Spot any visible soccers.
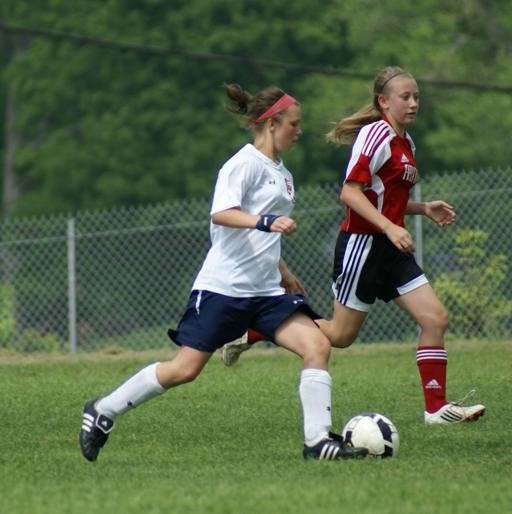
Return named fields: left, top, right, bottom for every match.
left=343, top=410, right=401, bottom=461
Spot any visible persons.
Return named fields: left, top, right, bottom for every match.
left=79, top=81, right=369, bottom=464
left=218, top=64, right=487, bottom=426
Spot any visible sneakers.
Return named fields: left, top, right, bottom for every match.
left=303, top=431, right=369, bottom=461
left=423, top=402, right=486, bottom=427
left=222, top=330, right=249, bottom=367
left=79, top=396, right=114, bottom=463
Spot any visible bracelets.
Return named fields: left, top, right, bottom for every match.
left=256, top=213, right=280, bottom=231
